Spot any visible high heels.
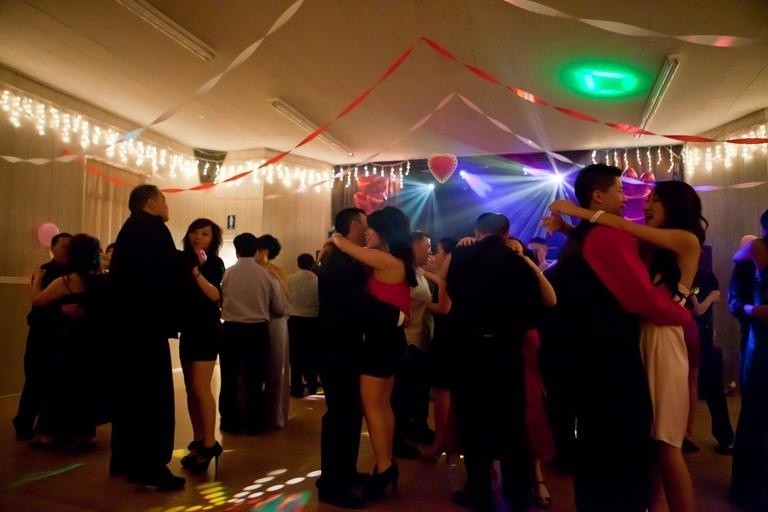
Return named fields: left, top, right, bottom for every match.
left=189, top=442, right=223, bottom=473
left=421, top=434, right=457, bottom=466
left=181, top=440, right=201, bottom=464
left=364, top=462, right=400, bottom=497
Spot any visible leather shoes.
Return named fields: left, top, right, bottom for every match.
left=127, top=466, right=186, bottom=491
left=683, top=432, right=700, bottom=453
left=345, top=471, right=371, bottom=487
left=535, top=480, right=551, bottom=507
left=718, top=432, right=735, bottom=454
left=391, top=436, right=420, bottom=458
left=318, top=490, right=367, bottom=509
left=451, top=489, right=488, bottom=510
left=13, top=417, right=97, bottom=455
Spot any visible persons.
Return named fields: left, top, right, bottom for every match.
left=287, top=161, right=766, bottom=512
left=11, top=185, right=287, bottom=488
